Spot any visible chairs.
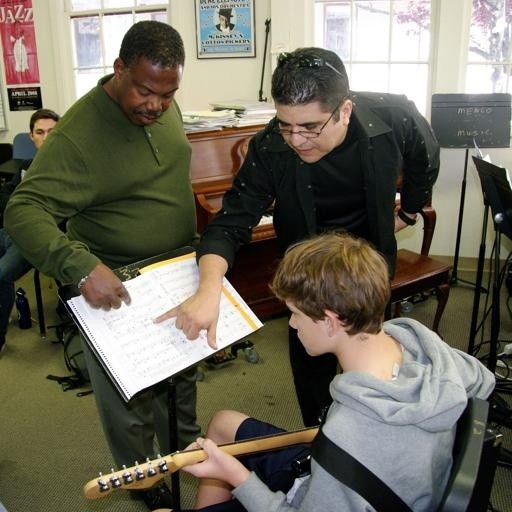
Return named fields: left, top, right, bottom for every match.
left=438, top=397, right=491, bottom=512
left=0, top=134, right=65, bottom=337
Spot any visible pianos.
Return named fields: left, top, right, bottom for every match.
left=184, top=123, right=436, bottom=321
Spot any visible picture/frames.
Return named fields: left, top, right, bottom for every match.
left=194, top=0, right=256, bottom=59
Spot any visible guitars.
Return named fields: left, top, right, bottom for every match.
left=81, top=424, right=321, bottom=499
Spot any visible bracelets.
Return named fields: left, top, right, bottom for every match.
left=397, top=210, right=418, bottom=229
left=76, top=274, right=90, bottom=290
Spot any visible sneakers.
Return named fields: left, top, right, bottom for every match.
left=139, top=483, right=174, bottom=509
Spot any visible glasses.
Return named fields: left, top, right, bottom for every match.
left=275, top=52, right=346, bottom=79
left=272, top=98, right=347, bottom=137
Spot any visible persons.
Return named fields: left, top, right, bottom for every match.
left=2, top=18, right=201, bottom=509
left=154, top=44, right=442, bottom=432
left=179, top=230, right=496, bottom=511
left=216, top=6, right=237, bottom=34
left=2, top=106, right=70, bottom=352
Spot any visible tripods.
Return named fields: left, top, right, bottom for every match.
left=486, top=213, right=512, bottom=428
left=431, top=149, right=489, bottom=293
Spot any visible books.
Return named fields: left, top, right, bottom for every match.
left=179, top=99, right=277, bottom=135
left=67, top=257, right=265, bottom=401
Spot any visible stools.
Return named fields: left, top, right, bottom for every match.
left=385, top=250, right=452, bottom=340
